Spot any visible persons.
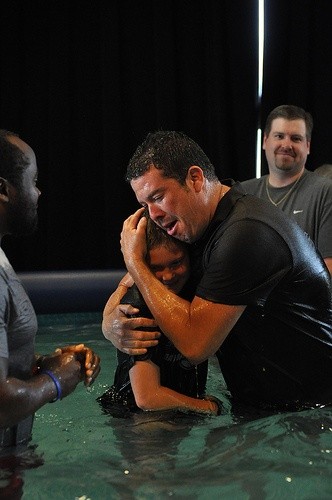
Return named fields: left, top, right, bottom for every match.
left=97, top=209, right=225, bottom=465
left=0, top=129, right=101, bottom=458
left=239, top=105, right=332, bottom=277
left=102, top=129, right=332, bottom=423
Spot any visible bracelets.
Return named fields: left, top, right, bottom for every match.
left=39, top=370, right=63, bottom=404
